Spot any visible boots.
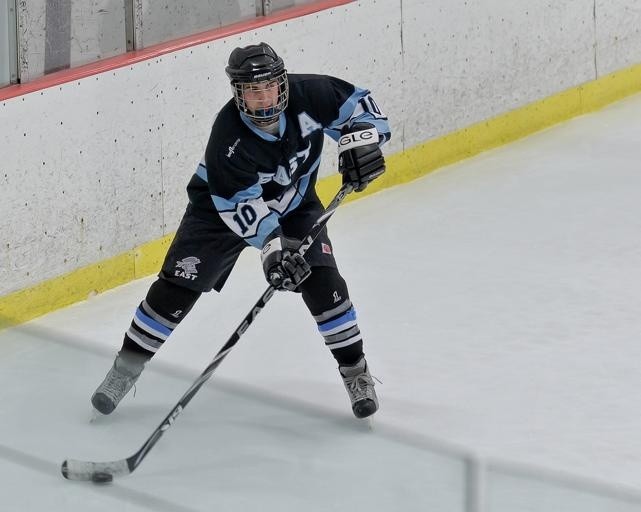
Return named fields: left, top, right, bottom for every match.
left=338, top=353, right=380, bottom=420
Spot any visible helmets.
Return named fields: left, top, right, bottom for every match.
left=225, top=41, right=284, bottom=82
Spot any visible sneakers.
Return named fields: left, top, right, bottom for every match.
left=91, top=356, right=145, bottom=415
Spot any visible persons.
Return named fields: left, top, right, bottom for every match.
left=91, top=39, right=393, bottom=420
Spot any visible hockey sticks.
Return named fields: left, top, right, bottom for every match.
left=62, top=176, right=355, bottom=482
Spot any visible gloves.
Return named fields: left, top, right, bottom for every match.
left=260, top=225, right=312, bottom=292
left=338, top=122, right=386, bottom=192
